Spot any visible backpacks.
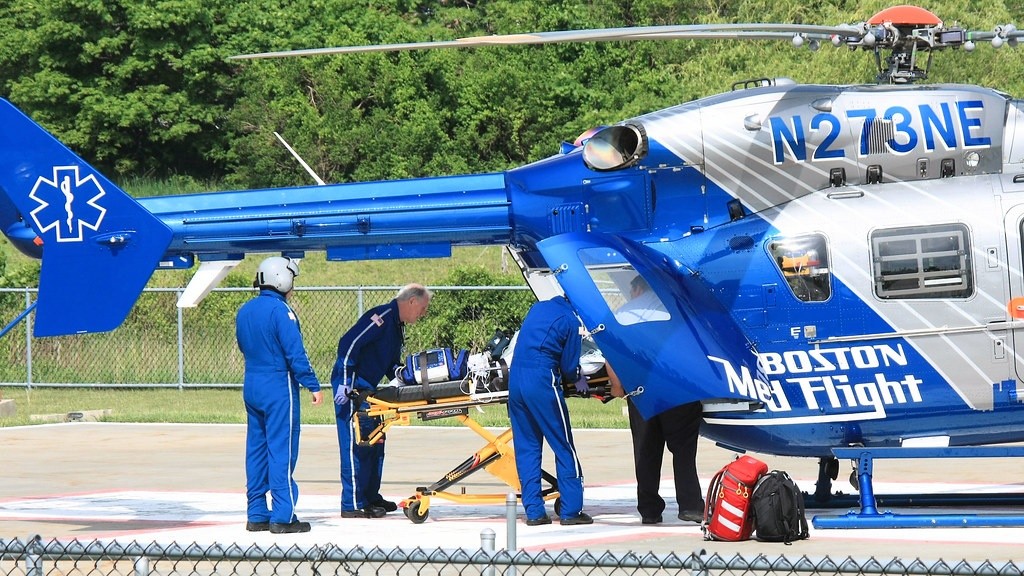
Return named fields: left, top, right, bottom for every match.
left=751, top=470, right=809, bottom=541
left=701, top=455, right=767, bottom=542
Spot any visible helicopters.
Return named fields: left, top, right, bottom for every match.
left=0, top=4, right=1024, bottom=529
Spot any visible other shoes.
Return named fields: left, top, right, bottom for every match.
left=641, top=515, right=662, bottom=524
left=247, top=521, right=268, bottom=531
left=678, top=509, right=711, bottom=523
left=270, top=520, right=311, bottom=533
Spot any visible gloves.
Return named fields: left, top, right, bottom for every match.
left=333, top=384, right=353, bottom=405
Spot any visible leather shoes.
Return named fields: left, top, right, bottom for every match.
left=560, top=513, right=594, bottom=525
left=341, top=505, right=385, bottom=518
left=527, top=514, right=552, bottom=525
left=372, top=499, right=397, bottom=512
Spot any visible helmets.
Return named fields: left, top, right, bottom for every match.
left=253, top=256, right=299, bottom=293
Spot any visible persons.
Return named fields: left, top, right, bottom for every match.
left=234, top=256, right=323, bottom=534
left=330, top=282, right=431, bottom=519
left=604, top=351, right=709, bottom=524
left=508, top=293, right=594, bottom=526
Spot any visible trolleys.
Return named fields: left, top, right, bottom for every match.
left=344, top=374, right=616, bottom=525
